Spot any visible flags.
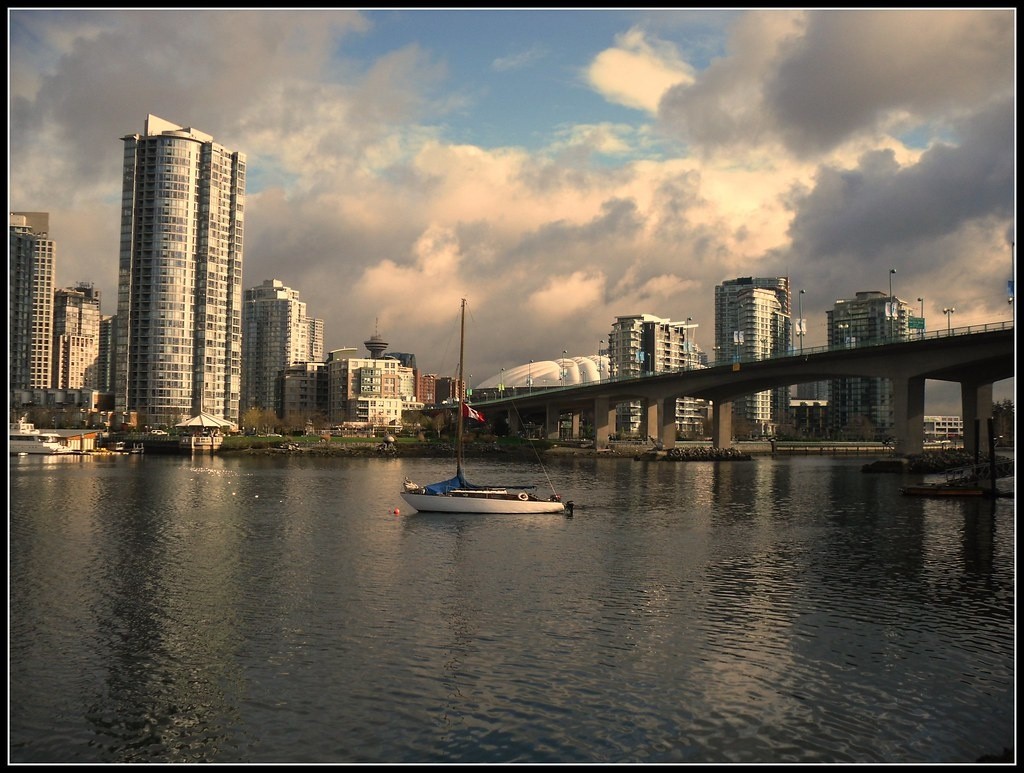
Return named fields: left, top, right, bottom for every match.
left=463, top=402, right=485, bottom=422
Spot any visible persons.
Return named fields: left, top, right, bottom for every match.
left=666, top=446, right=742, bottom=458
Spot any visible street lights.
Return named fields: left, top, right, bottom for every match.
left=761, top=339, right=767, bottom=360
left=838, top=321, right=849, bottom=349
left=501, top=368, right=505, bottom=400
left=561, top=350, right=567, bottom=386
left=1008, top=296, right=1014, bottom=322
left=889, top=269, right=897, bottom=342
left=529, top=360, right=533, bottom=396
left=799, top=289, right=806, bottom=355
left=712, top=346, right=720, bottom=366
left=581, top=370, right=586, bottom=385
left=686, top=317, right=692, bottom=371
left=600, top=340, right=604, bottom=384
left=917, top=298, right=924, bottom=340
left=737, top=304, right=743, bottom=363
left=943, top=307, right=955, bottom=336
left=638, top=329, right=644, bottom=379
left=468, top=375, right=472, bottom=404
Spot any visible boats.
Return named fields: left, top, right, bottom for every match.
left=10, top=416, right=73, bottom=454
left=899, top=416, right=1014, bottom=497
left=107, top=441, right=144, bottom=454
left=399, top=298, right=574, bottom=514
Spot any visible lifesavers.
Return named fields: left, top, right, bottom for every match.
left=518, top=492, right=528, bottom=501
left=668, top=447, right=741, bottom=459
